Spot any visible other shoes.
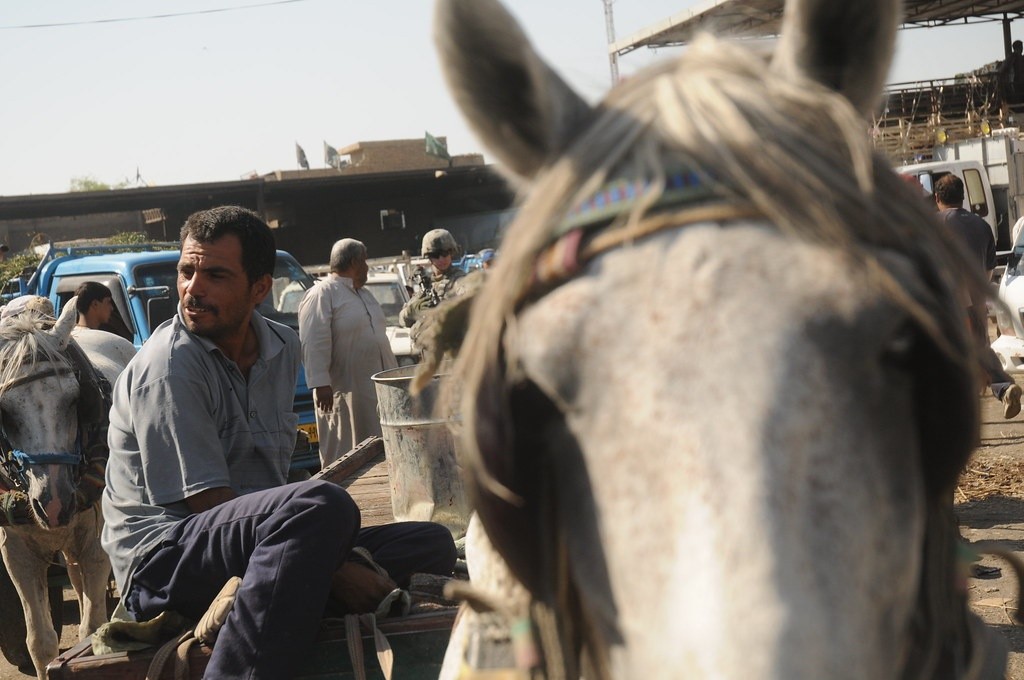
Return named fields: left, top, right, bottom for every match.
left=1000, top=384, right=1022, bottom=419
left=193, top=576, right=243, bottom=648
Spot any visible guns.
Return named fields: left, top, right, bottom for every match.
left=410, top=267, right=441, bottom=307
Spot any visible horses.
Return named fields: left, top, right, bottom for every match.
left=0, top=292, right=138, bottom=680
left=420, top=0, right=1007, bottom=679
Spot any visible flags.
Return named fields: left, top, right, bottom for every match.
left=425, top=135, right=452, bottom=160
left=324, top=144, right=342, bottom=174
left=296, top=145, right=310, bottom=171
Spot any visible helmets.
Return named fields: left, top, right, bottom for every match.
left=479, top=249, right=495, bottom=268
left=421, top=228, right=458, bottom=259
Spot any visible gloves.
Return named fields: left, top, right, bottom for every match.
left=404, top=290, right=434, bottom=320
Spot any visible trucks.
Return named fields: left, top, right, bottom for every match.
left=0, top=240, right=321, bottom=480
left=320, top=254, right=502, bottom=366
left=890, top=134, right=1024, bottom=275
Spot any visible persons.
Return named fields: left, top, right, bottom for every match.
left=482, top=252, right=496, bottom=272
left=1011, top=39, right=1024, bottom=113
left=297, top=237, right=398, bottom=472
left=399, top=228, right=468, bottom=327
left=99, top=205, right=459, bottom=680
left=72, top=281, right=114, bottom=330
left=932, top=173, right=1024, bottom=419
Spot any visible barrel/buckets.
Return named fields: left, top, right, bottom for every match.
left=371, top=358, right=473, bottom=542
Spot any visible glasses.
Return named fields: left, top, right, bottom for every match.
left=427, top=248, right=452, bottom=259
